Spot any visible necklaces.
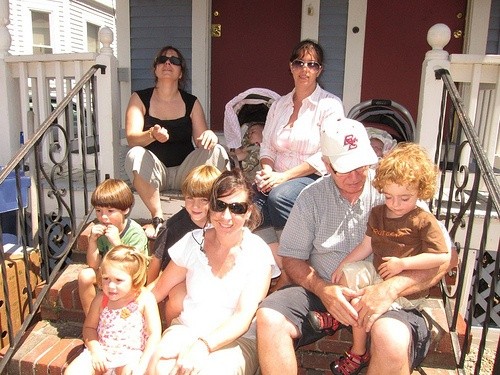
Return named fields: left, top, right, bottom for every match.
left=191, top=220, right=208, bottom=251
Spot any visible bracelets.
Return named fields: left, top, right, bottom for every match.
left=196, top=335, right=213, bottom=351
left=148, top=132, right=152, bottom=138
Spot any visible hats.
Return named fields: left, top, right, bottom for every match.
left=320, top=116, right=378, bottom=173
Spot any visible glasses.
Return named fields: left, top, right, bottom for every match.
left=210, top=200, right=248, bottom=214
left=291, top=60, right=322, bottom=71
left=330, top=164, right=371, bottom=177
left=154, top=56, right=182, bottom=67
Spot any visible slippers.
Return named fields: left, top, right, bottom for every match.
left=145, top=217, right=165, bottom=240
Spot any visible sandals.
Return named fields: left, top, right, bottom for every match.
left=306, top=311, right=341, bottom=336
left=330, top=346, right=368, bottom=375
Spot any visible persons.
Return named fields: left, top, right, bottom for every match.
left=248, top=40, right=345, bottom=292
left=146, top=170, right=281, bottom=375
left=234, top=123, right=385, bottom=168
left=77, top=164, right=221, bottom=318
left=307, top=143, right=449, bottom=375
left=257, top=117, right=459, bottom=375
left=124, top=46, right=229, bottom=239
left=64, top=246, right=161, bottom=375
